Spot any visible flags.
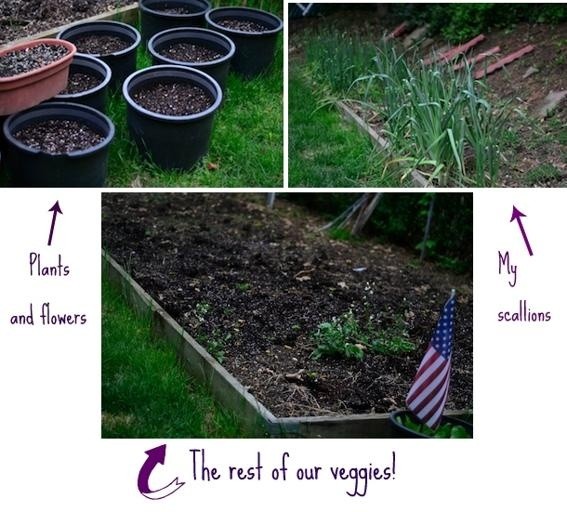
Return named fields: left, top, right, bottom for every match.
left=405, top=295, right=458, bottom=434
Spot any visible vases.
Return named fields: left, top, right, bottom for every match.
left=133, top=2, right=213, bottom=50
left=0, top=39, right=74, bottom=115
left=58, top=17, right=143, bottom=86
left=4, top=104, right=116, bottom=188
left=203, top=4, right=282, bottom=82
left=50, top=53, right=113, bottom=122
left=142, top=28, right=236, bottom=93
left=118, top=61, right=222, bottom=169
